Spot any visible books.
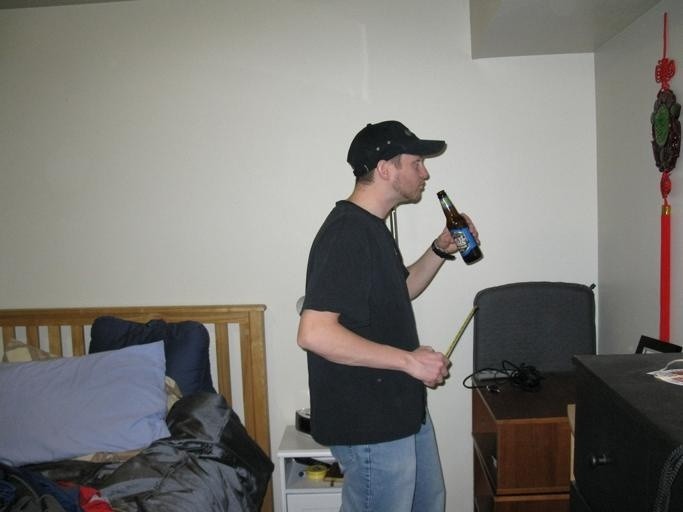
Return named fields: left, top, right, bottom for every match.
left=323, top=461, right=345, bottom=483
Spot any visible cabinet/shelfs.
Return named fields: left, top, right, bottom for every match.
left=573, top=352, right=681, bottom=511
left=470, top=377, right=576, bottom=512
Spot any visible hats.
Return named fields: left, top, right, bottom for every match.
left=347, top=120, right=445, bottom=177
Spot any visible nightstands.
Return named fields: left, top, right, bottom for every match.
left=273, top=423, right=345, bottom=511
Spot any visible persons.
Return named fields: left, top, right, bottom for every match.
left=296, top=118, right=482, bottom=512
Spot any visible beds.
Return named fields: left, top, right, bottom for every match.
left=0, top=304, right=274, bottom=511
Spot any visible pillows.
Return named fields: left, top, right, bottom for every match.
left=0, top=339, right=172, bottom=468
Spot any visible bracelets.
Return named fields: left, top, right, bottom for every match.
left=428, top=238, right=458, bottom=265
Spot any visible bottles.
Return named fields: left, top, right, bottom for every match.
left=437, top=189, right=485, bottom=266
